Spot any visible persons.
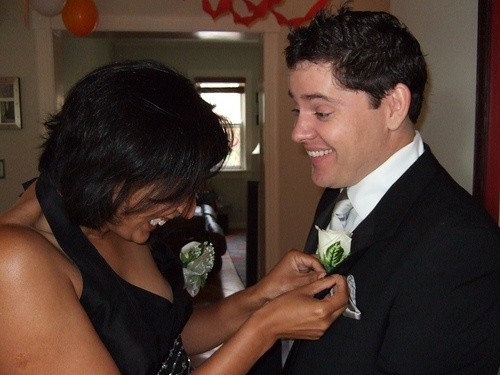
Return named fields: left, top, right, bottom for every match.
left=0, top=59, right=350, bottom=375
left=246, top=1, right=500, bottom=375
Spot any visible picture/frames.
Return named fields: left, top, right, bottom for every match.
left=0, top=76, right=22, bottom=130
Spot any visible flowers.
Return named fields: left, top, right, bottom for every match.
left=314, top=225, right=354, bottom=273
left=180, top=241, right=215, bottom=297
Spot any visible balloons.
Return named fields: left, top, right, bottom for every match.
left=29, top=0, right=99, bottom=37
